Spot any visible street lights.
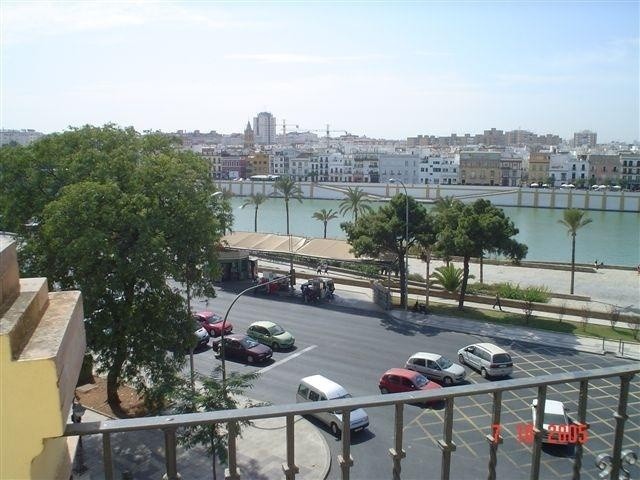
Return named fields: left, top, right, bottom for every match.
left=389, top=179, right=408, bottom=286
left=186, top=191, right=222, bottom=412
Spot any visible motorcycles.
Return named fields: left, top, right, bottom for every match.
left=412, top=300, right=427, bottom=314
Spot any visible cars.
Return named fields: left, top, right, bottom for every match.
left=378, top=352, right=467, bottom=406
left=531, top=398, right=570, bottom=449
left=191, top=311, right=295, bottom=364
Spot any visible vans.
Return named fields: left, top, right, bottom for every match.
left=296, top=374, right=369, bottom=435
left=301, top=278, right=335, bottom=293
left=457, top=343, right=513, bottom=379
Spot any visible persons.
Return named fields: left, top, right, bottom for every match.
left=301, top=283, right=336, bottom=305
left=594, top=260, right=598, bottom=269
left=324, top=263, right=329, bottom=274
left=317, top=264, right=321, bottom=274
left=637, top=265, right=640, bottom=276
left=493, top=292, right=502, bottom=311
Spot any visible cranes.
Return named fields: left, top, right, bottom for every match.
left=316, top=124, right=348, bottom=148
left=264, top=118, right=299, bottom=146
